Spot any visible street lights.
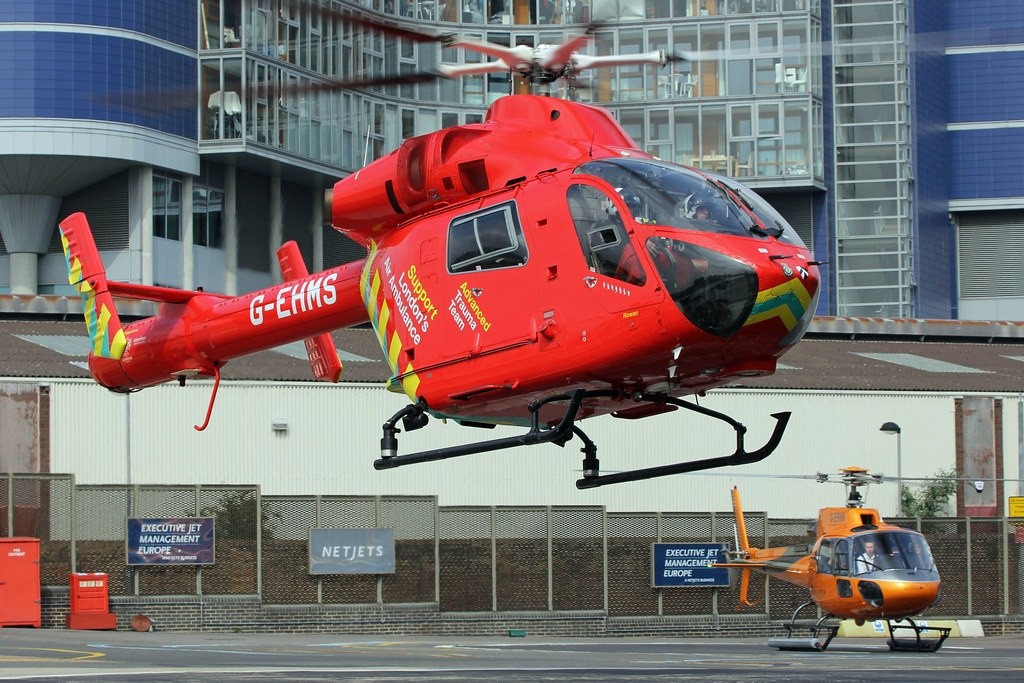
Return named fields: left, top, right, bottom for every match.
left=880, top=421, right=903, bottom=520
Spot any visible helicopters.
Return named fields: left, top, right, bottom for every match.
left=684, top=455, right=1024, bottom=653
left=58, top=9, right=823, bottom=489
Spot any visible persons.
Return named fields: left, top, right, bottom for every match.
left=856, top=540, right=880, bottom=575
left=682, top=191, right=718, bottom=222
left=588, top=184, right=697, bottom=291
